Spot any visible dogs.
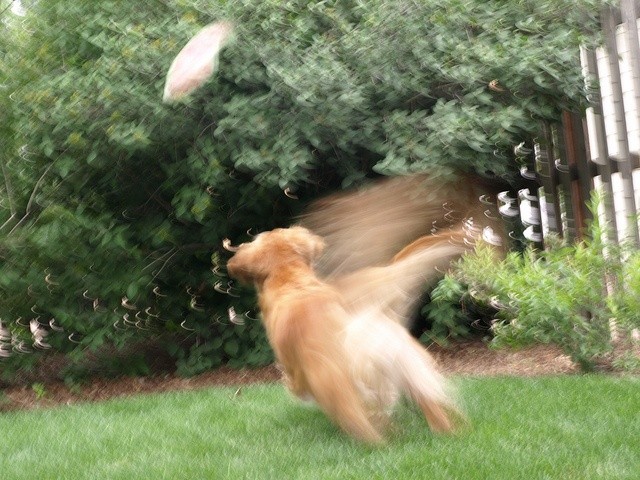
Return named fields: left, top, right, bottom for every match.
left=222, top=223, right=472, bottom=448
